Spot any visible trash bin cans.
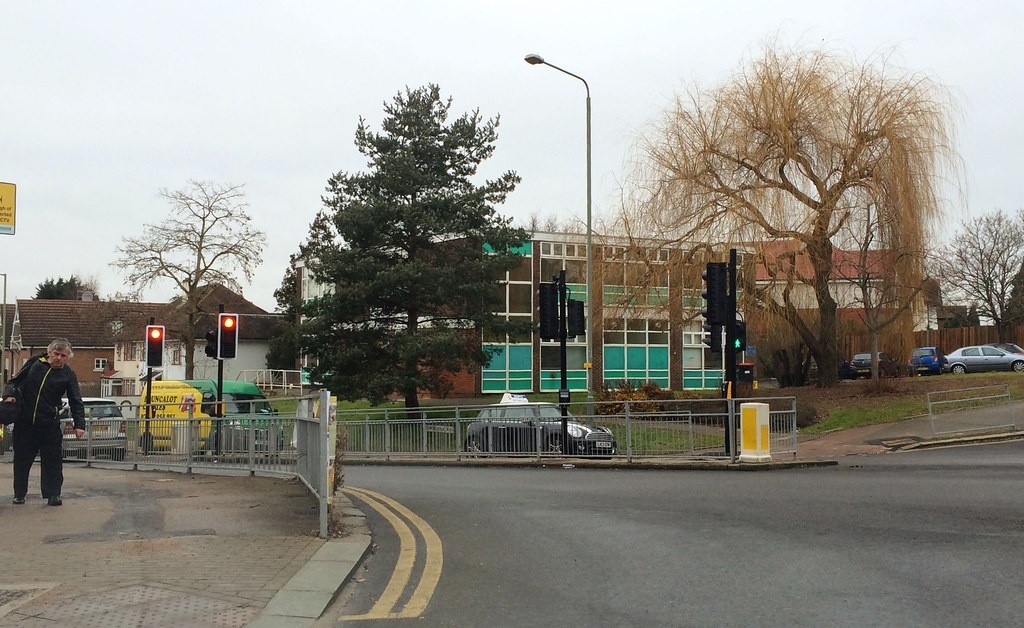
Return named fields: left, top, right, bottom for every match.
left=171, top=423, right=189, bottom=461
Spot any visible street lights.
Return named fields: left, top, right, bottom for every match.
left=524, top=52, right=594, bottom=425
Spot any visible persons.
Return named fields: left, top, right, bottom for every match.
left=0, top=337, right=86, bottom=506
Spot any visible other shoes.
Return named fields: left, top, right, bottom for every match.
left=47, top=495, right=62, bottom=505
left=13, top=497, right=26, bottom=504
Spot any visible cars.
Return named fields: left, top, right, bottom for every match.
left=802, top=353, right=858, bottom=380
left=943, top=345, right=1024, bottom=375
left=463, top=401, right=618, bottom=460
left=906, top=346, right=945, bottom=377
left=983, top=342, right=1024, bottom=360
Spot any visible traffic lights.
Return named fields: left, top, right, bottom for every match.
left=702, top=324, right=724, bottom=353
left=566, top=295, right=586, bottom=339
left=699, top=261, right=731, bottom=325
left=205, top=328, right=218, bottom=358
left=145, top=324, right=166, bottom=369
left=216, top=312, right=240, bottom=360
left=731, top=320, right=748, bottom=354
left=536, top=281, right=560, bottom=342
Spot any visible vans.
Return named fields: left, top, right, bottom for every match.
left=138, top=379, right=284, bottom=460
left=850, top=351, right=900, bottom=379
left=59, top=397, right=128, bottom=461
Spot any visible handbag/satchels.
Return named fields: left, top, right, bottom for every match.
left=31, top=419, right=61, bottom=441
